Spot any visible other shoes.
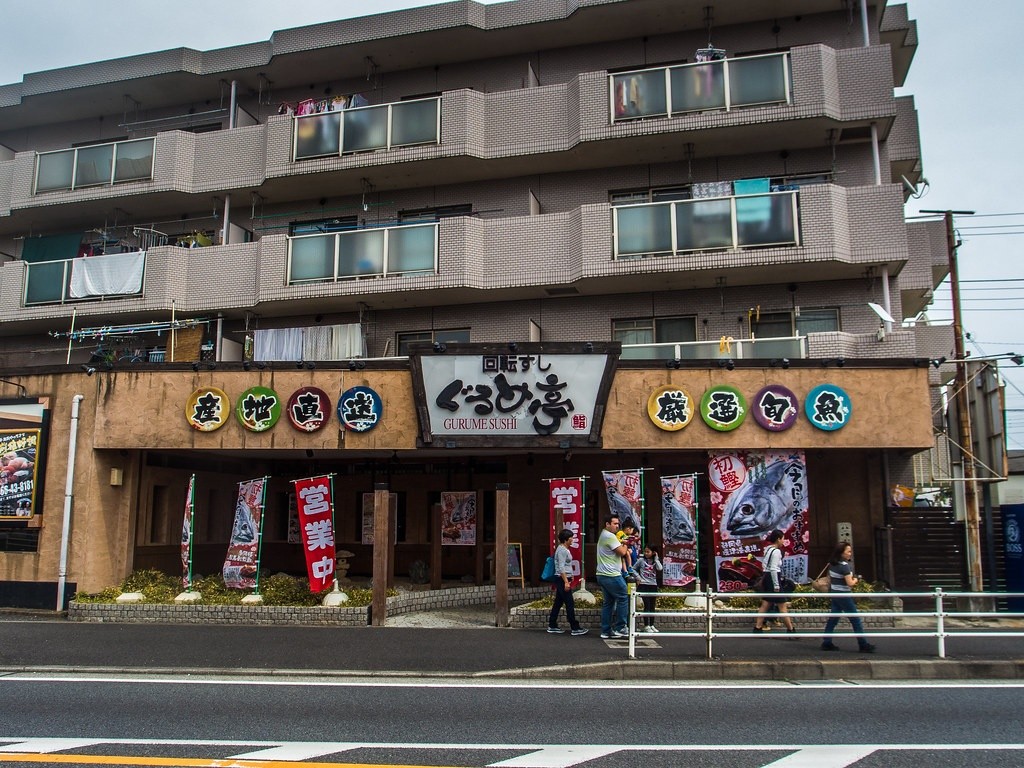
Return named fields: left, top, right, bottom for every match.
left=651, top=625, right=659, bottom=633
left=571, top=628, right=589, bottom=635
left=623, top=571, right=630, bottom=579
left=600, top=632, right=621, bottom=638
left=859, top=642, right=877, bottom=652
left=615, top=626, right=629, bottom=636
left=644, top=626, right=654, bottom=632
left=547, top=628, right=565, bottom=633
left=627, top=566, right=634, bottom=573
left=823, top=643, right=839, bottom=652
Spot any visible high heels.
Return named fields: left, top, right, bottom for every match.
left=753, top=626, right=763, bottom=633
left=787, top=627, right=800, bottom=640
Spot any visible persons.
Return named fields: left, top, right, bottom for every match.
left=630, top=544, right=663, bottom=633
left=596, top=514, right=636, bottom=638
left=753, top=530, right=801, bottom=634
left=547, top=529, right=589, bottom=636
left=821, top=542, right=878, bottom=653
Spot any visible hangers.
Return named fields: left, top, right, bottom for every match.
left=282, top=93, right=363, bottom=107
left=694, top=43, right=727, bottom=61
left=124, top=348, right=145, bottom=362
left=87, top=223, right=169, bottom=247
left=92, top=345, right=120, bottom=364
left=179, top=228, right=214, bottom=241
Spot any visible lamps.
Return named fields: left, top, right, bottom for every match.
left=931, top=356, right=947, bottom=369
left=82, top=364, right=101, bottom=377
left=433, top=340, right=594, bottom=353
left=192, top=360, right=367, bottom=373
left=666, top=356, right=848, bottom=371
left=962, top=352, right=1023, bottom=366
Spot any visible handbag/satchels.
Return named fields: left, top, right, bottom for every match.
left=541, top=556, right=555, bottom=581
left=812, top=563, right=831, bottom=592
left=753, top=575, right=764, bottom=591
